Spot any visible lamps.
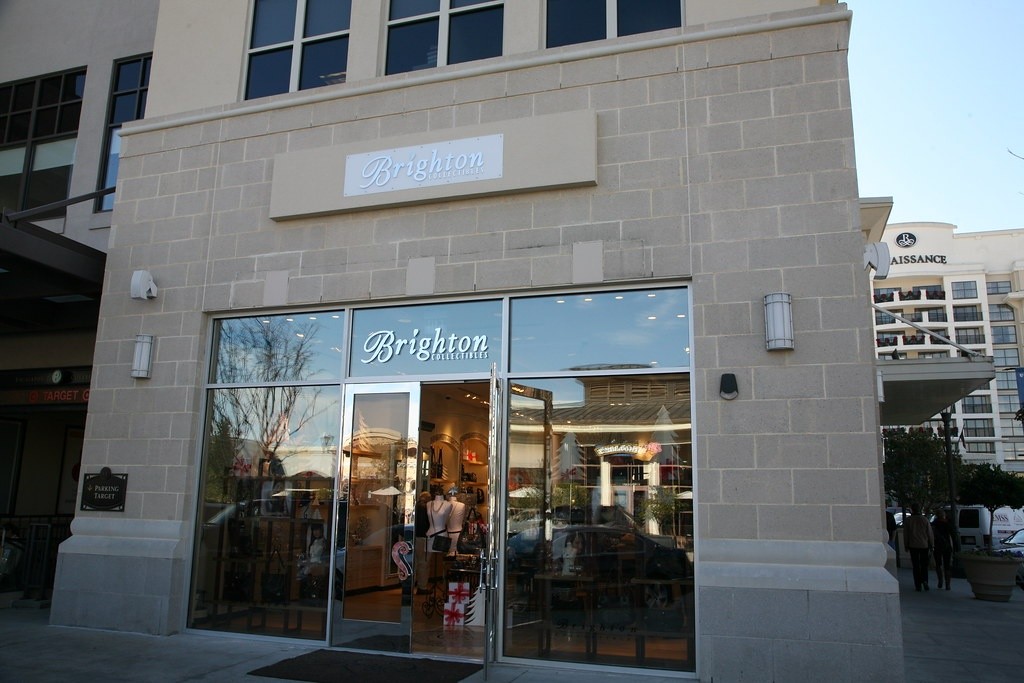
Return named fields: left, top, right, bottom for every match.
left=130, top=333, right=154, bottom=379
left=763, top=292, right=794, bottom=351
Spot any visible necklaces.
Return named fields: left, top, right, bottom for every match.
left=433, top=501, right=444, bottom=512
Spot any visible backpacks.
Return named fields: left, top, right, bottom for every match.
left=477, top=488, right=484, bottom=504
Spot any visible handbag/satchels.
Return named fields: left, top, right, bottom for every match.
left=422, top=484, right=445, bottom=500
left=455, top=507, right=487, bottom=554
left=431, top=448, right=449, bottom=481
left=432, top=535, right=452, bottom=553
left=467, top=555, right=480, bottom=564
left=452, top=560, right=467, bottom=569
left=461, top=471, right=477, bottom=482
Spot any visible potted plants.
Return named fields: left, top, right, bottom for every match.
left=958, top=545, right=1024, bottom=602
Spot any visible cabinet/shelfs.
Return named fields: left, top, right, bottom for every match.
left=256, top=429, right=488, bottom=641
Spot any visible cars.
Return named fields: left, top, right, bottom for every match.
left=203, top=496, right=292, bottom=575
left=507, top=523, right=689, bottom=624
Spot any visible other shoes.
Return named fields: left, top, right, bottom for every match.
left=923, top=584, right=929, bottom=591
left=937, top=580, right=944, bottom=588
left=945, top=582, right=951, bottom=589
left=916, top=584, right=922, bottom=591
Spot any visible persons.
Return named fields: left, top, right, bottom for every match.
left=261, top=478, right=289, bottom=517
left=414, top=491, right=433, bottom=595
left=930, top=510, right=960, bottom=590
left=477, top=489, right=485, bottom=504
left=551, top=531, right=587, bottom=579
left=903, top=504, right=935, bottom=593
left=298, top=499, right=322, bottom=519
left=297, top=522, right=328, bottom=608
left=885, top=501, right=896, bottom=550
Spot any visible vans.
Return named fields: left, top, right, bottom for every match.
left=931, top=506, right=1023, bottom=578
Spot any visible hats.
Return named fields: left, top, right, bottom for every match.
left=934, top=509, right=945, bottom=516
left=310, top=523, right=324, bottom=540
left=910, top=502, right=923, bottom=508
